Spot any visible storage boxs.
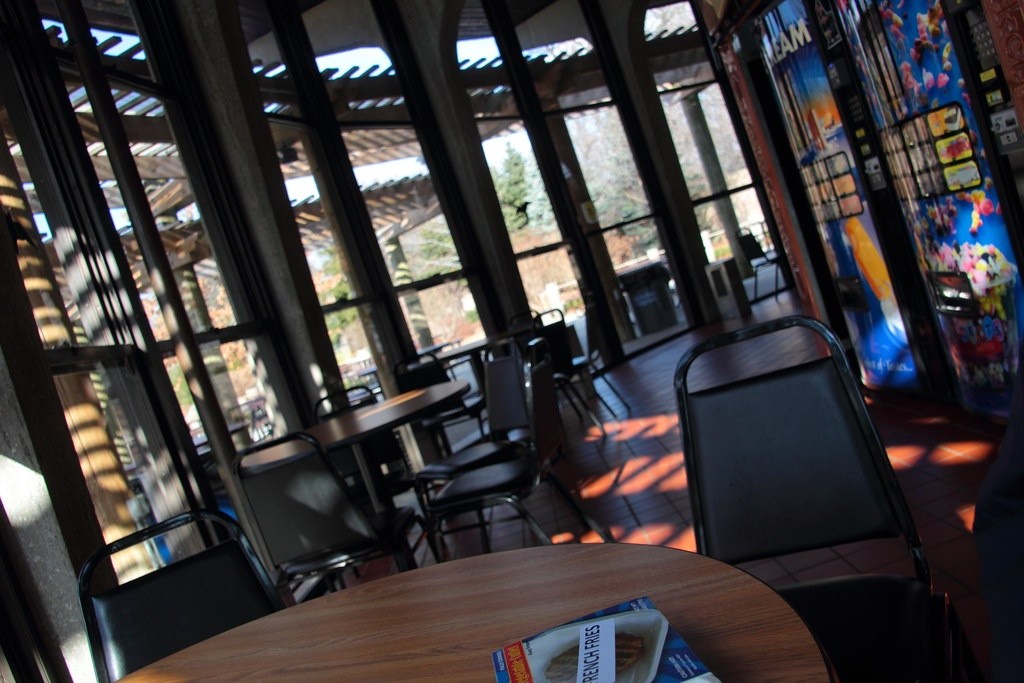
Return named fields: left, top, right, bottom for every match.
left=490, top=593, right=720, bottom=683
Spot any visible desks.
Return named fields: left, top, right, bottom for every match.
left=424, top=324, right=535, bottom=393
left=117, top=544, right=841, bottom=682
left=359, top=343, right=454, bottom=399
left=239, top=379, right=475, bottom=572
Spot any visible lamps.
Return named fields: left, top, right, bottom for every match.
left=277, top=133, right=301, bottom=166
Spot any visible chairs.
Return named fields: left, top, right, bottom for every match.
left=736, top=227, right=780, bottom=303
left=74, top=309, right=987, bottom=683
